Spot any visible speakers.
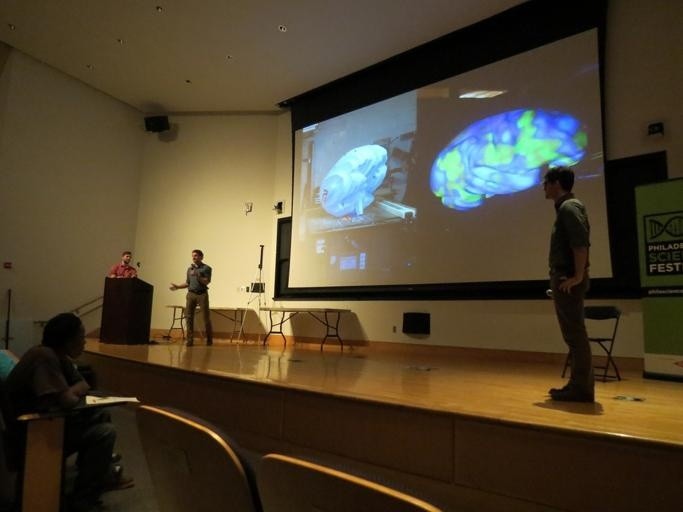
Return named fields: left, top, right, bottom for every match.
left=403, top=312, right=430, bottom=335
left=144, top=116, right=169, bottom=132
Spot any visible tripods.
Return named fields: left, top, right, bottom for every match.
left=236, top=268, right=270, bottom=346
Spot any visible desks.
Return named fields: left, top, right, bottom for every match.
left=259, top=307, right=352, bottom=354
left=15, top=390, right=141, bottom=512
left=168, top=305, right=255, bottom=344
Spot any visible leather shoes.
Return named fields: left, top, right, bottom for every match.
left=112, top=453, right=122, bottom=463
left=113, top=465, right=123, bottom=476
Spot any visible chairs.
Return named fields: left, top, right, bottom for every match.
left=560, top=306, right=623, bottom=383
left=256, top=453, right=442, bottom=511
left=137, top=404, right=261, bottom=512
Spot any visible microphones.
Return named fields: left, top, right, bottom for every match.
left=133, top=263, right=140, bottom=278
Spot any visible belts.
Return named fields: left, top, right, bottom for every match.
left=188, top=290, right=203, bottom=293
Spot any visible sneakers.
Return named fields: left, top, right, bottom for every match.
left=549, top=378, right=595, bottom=402
left=186, top=341, right=193, bottom=347
left=206, top=339, right=212, bottom=346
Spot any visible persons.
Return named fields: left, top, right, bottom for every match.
left=1, top=313, right=123, bottom=494
left=543, top=167, right=594, bottom=403
left=169, top=250, right=212, bottom=346
left=108, top=251, right=138, bottom=278
